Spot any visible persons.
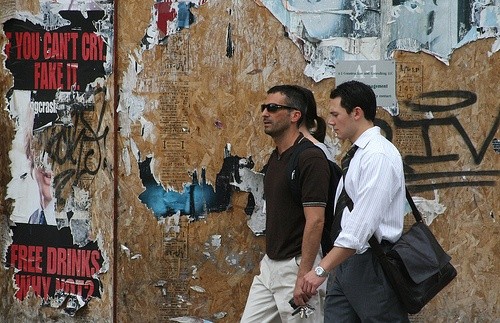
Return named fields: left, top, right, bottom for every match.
left=240, top=85, right=331, bottom=323
left=300, top=80, right=409, bottom=323
left=25, top=112, right=58, bottom=226
left=295, top=85, right=335, bottom=163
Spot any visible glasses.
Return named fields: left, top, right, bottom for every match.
left=260, top=102, right=297, bottom=112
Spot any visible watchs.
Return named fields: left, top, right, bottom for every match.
left=314, top=265, right=329, bottom=277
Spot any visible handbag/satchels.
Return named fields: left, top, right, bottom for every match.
left=377, top=219, right=457, bottom=314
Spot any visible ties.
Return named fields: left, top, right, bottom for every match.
left=330, top=145, right=359, bottom=244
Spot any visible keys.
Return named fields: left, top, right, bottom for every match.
left=291, top=305, right=316, bottom=319
